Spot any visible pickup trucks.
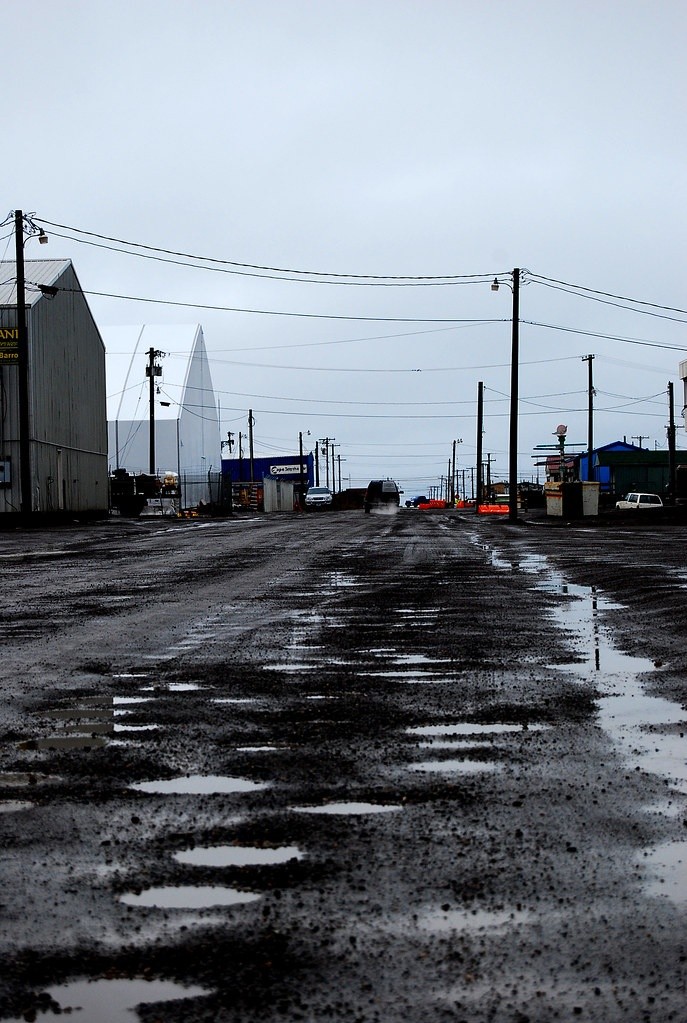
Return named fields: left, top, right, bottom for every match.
left=405, top=495, right=435, bottom=508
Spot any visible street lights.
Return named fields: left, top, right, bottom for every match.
left=13, top=232, right=50, bottom=526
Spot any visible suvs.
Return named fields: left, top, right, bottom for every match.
left=303, top=486, right=333, bottom=509
left=615, top=493, right=664, bottom=508
left=363, top=480, right=405, bottom=513
